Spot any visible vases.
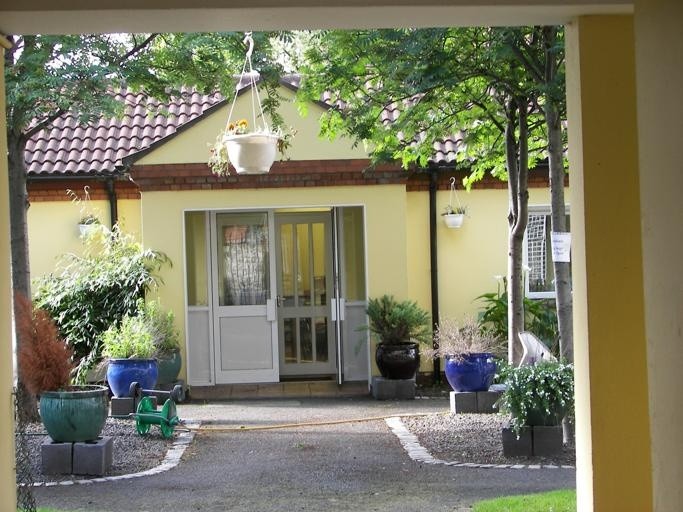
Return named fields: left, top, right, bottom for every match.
left=444, top=352, right=496, bottom=392
left=224, top=133, right=280, bottom=175
left=524, top=406, right=566, bottom=426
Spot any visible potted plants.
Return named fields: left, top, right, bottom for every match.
left=97, top=296, right=182, bottom=397
left=78, top=213, right=100, bottom=235
left=13, top=288, right=109, bottom=442
left=441, top=205, right=466, bottom=228
left=364, top=294, right=432, bottom=379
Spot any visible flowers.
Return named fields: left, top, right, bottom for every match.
left=487, top=357, right=575, bottom=441
left=417, top=312, right=509, bottom=366
left=205, top=119, right=299, bottom=178
